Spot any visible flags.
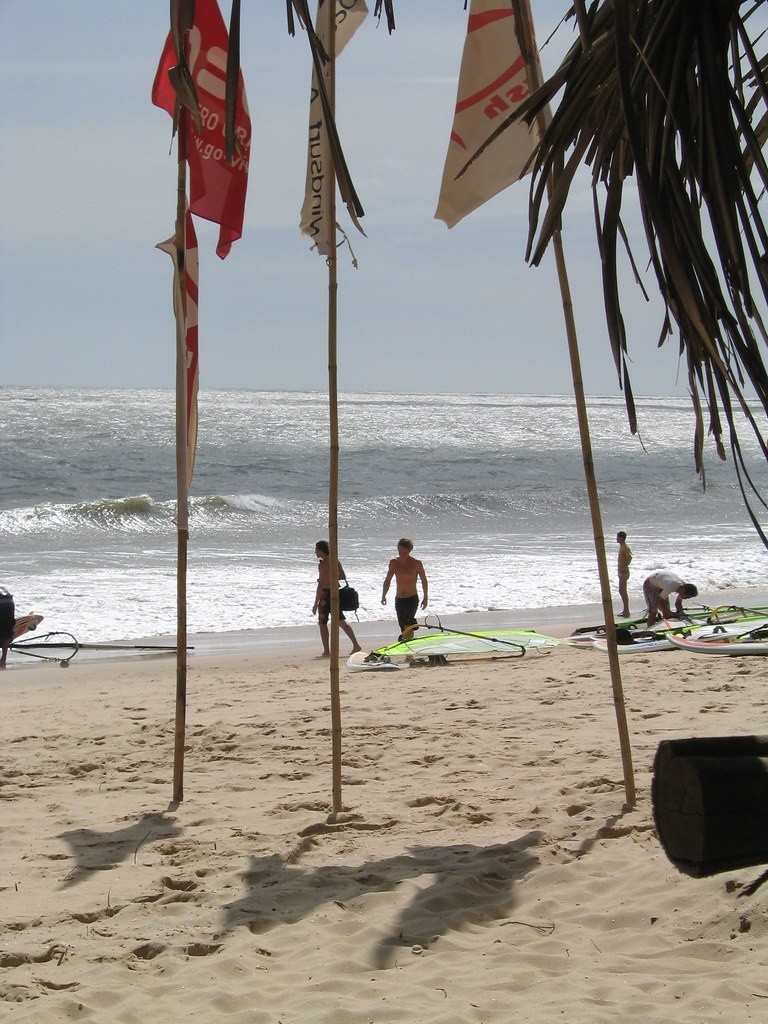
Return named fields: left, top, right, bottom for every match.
left=299, top=0, right=369, bottom=254
left=156, top=193, right=199, bottom=490
left=151, top=0, right=251, bottom=259
left=434, top=0, right=556, bottom=229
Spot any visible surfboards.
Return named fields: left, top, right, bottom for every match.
left=666, top=633, right=768, bottom=656
left=13, top=614, right=44, bottom=638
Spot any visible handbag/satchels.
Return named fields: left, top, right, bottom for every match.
left=339, top=579, right=359, bottom=610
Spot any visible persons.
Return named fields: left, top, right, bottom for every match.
left=643, top=571, right=698, bottom=628
left=381, top=537, right=428, bottom=662
left=0, top=586, right=15, bottom=669
left=616, top=531, right=633, bottom=617
left=312, top=541, right=362, bottom=658
